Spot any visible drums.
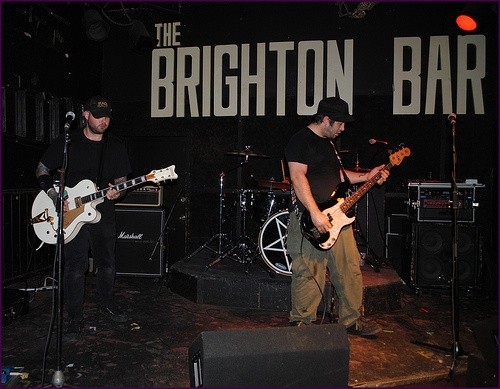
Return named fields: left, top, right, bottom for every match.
left=263, top=189, right=292, bottom=224
left=256, top=209, right=292, bottom=276
left=218, top=188, right=259, bottom=258
left=257, top=173, right=291, bottom=191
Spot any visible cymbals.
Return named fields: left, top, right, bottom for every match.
left=337, top=148, right=371, bottom=173
left=224, top=149, right=270, bottom=157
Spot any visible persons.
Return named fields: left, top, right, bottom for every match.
left=35, top=96, right=140, bottom=341
left=288, top=97, right=391, bottom=338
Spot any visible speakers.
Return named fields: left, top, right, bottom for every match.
left=186, top=324, right=351, bottom=389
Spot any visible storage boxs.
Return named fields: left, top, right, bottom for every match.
left=113, top=208, right=168, bottom=277
left=410, top=223, right=483, bottom=289
left=406, top=179, right=484, bottom=211
left=383, top=233, right=412, bottom=267
left=387, top=211, right=409, bottom=235
left=416, top=181, right=477, bottom=223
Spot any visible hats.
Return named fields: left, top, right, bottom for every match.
left=85, top=95, right=112, bottom=118
left=317, top=97, right=354, bottom=123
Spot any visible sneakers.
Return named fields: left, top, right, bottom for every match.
left=97, top=300, right=128, bottom=323
left=65, top=316, right=83, bottom=341
left=348, top=317, right=384, bottom=337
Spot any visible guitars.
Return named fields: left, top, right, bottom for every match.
left=300, top=143, right=412, bottom=251
left=31, top=164, right=178, bottom=245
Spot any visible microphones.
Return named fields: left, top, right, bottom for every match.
left=63, top=110, right=76, bottom=130
left=448, top=112, right=458, bottom=124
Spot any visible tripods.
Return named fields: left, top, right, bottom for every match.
left=38, top=133, right=79, bottom=389
left=409, top=127, right=482, bottom=379
